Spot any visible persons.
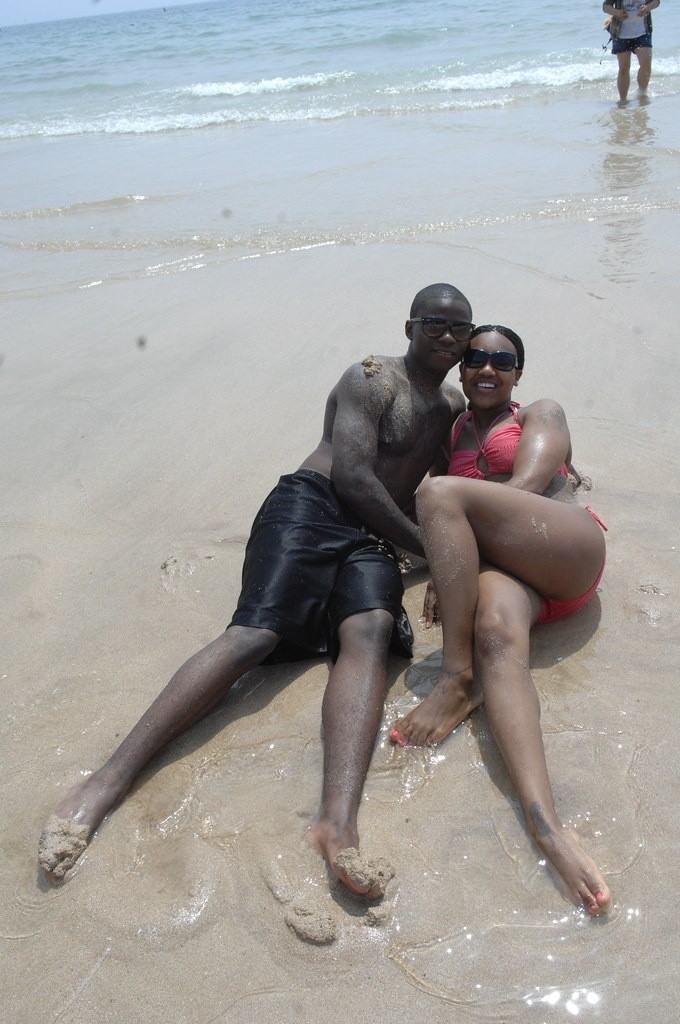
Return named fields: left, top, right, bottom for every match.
left=603, top=0, right=660, bottom=99
left=391, top=324, right=609, bottom=913
left=38, top=283, right=472, bottom=899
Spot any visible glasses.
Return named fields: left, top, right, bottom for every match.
left=461, top=347, right=520, bottom=374
left=409, top=315, right=475, bottom=343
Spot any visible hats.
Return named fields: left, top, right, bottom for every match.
left=603, top=18, right=612, bottom=30
